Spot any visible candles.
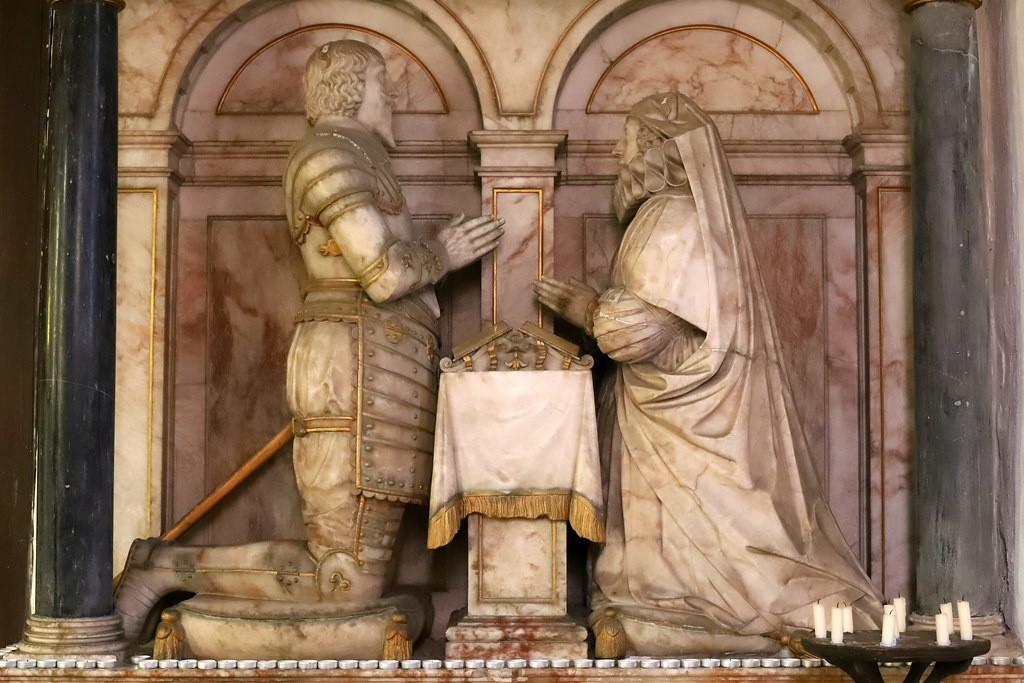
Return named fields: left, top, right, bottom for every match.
left=830, top=608, right=842, bottom=643
left=883, top=604, right=901, bottom=637
left=842, top=605, right=854, bottom=632
left=941, top=601, right=953, bottom=632
left=812, top=598, right=827, bottom=637
left=880, top=615, right=897, bottom=647
left=958, top=596, right=972, bottom=641
left=934, top=613, right=950, bottom=647
left=896, top=595, right=907, bottom=631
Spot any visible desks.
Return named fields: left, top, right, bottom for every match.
left=801, top=629, right=989, bottom=683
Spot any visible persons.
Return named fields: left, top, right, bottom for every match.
left=532, top=90, right=886, bottom=633
left=113, top=37, right=508, bottom=645
